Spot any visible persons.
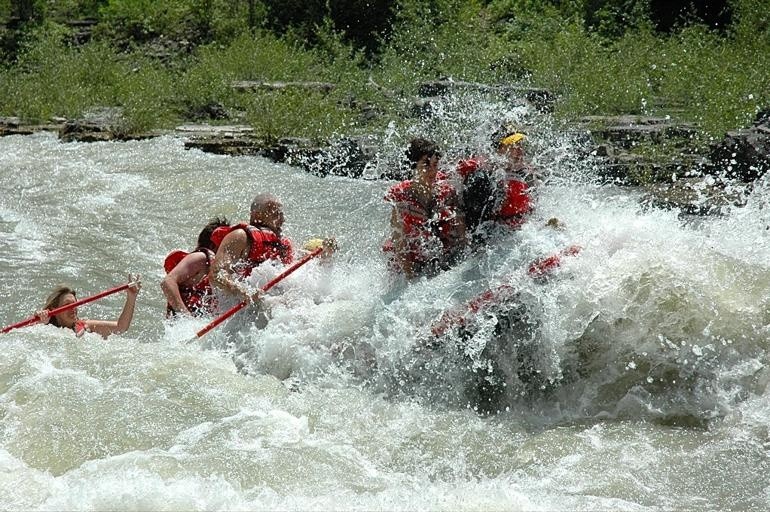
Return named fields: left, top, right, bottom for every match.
left=29, top=270, right=138, bottom=342
left=456, top=135, right=556, bottom=256
left=204, top=188, right=336, bottom=330
left=377, top=137, right=478, bottom=280
left=160, top=215, right=228, bottom=328
